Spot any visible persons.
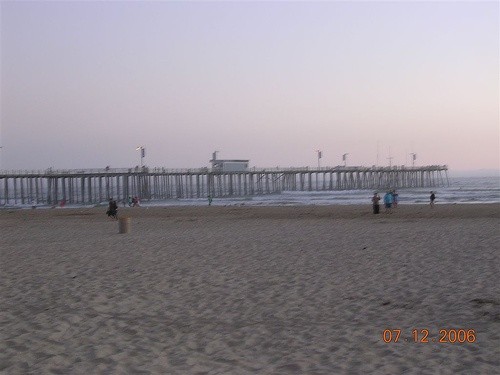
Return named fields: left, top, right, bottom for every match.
left=390, top=187, right=398, bottom=207
left=31, top=197, right=37, bottom=210
left=52, top=198, right=56, bottom=209
left=429, top=190, right=435, bottom=207
left=383, top=190, right=394, bottom=214
left=207, top=194, right=213, bottom=209
left=371, top=191, right=381, bottom=215
left=128, top=193, right=140, bottom=208
left=107, top=199, right=120, bottom=221
left=60, top=198, right=64, bottom=208
